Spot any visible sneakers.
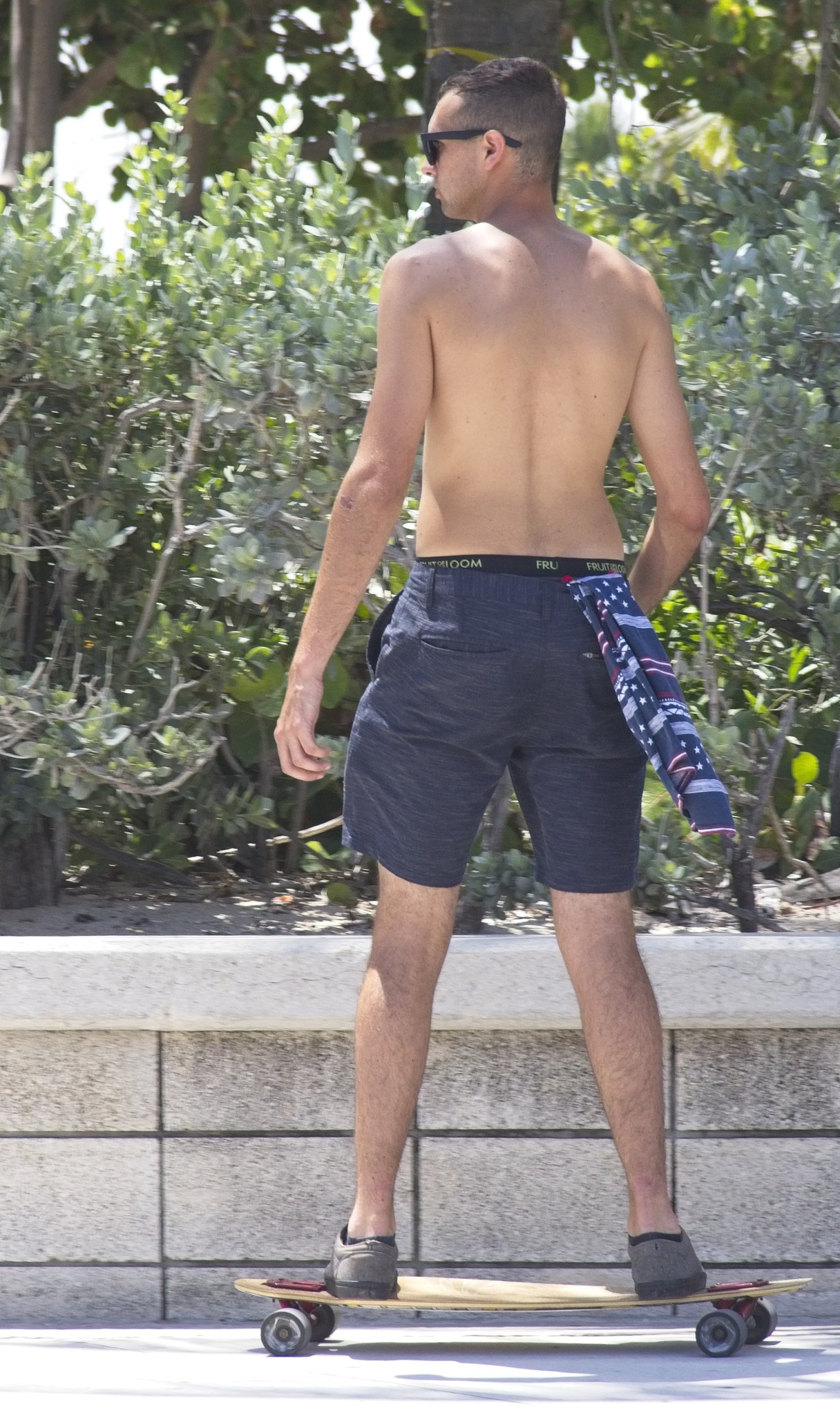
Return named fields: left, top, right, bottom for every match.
left=325, top=1225, right=399, bottom=1301
left=627, top=1231, right=707, bottom=1299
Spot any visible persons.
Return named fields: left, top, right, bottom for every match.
left=266, top=55, right=720, bottom=1299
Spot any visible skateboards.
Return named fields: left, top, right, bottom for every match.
left=235, top=1275, right=816, bottom=1356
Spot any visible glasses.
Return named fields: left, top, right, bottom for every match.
left=420, top=129, right=523, bottom=166
